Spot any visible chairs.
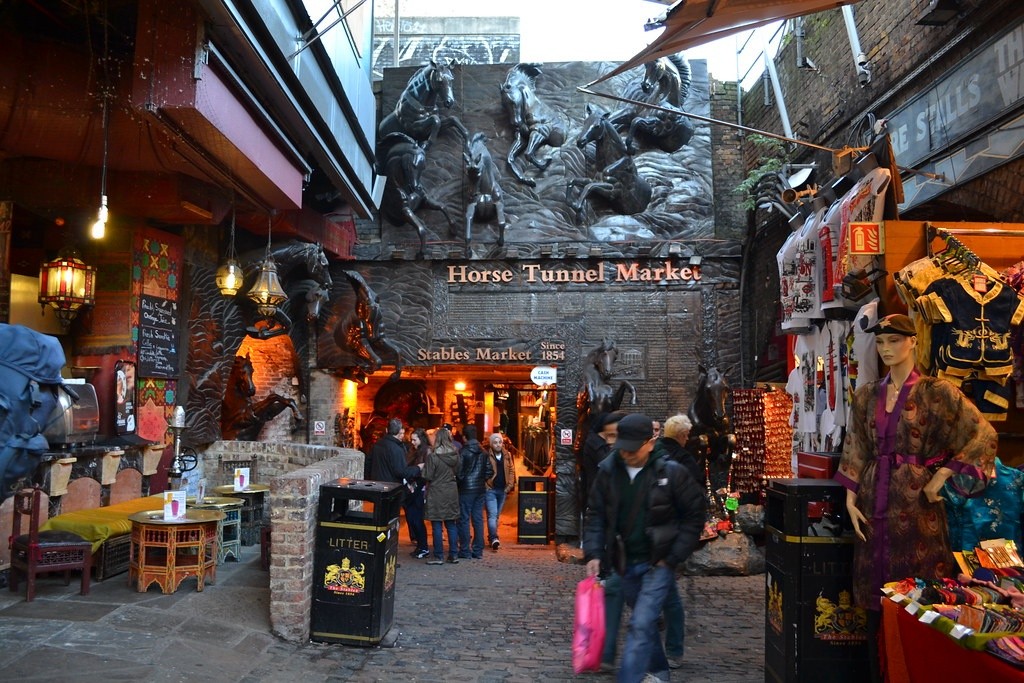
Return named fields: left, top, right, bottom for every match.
left=260, top=526, right=270, bottom=571
left=11, top=482, right=92, bottom=602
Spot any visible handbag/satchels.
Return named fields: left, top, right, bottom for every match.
left=573, top=573, right=606, bottom=674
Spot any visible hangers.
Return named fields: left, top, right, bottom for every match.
left=934, top=232, right=996, bottom=284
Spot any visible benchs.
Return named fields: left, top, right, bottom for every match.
left=39, top=493, right=166, bottom=581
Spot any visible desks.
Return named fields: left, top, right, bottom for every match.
left=127, top=484, right=270, bottom=595
left=797, top=451, right=842, bottom=479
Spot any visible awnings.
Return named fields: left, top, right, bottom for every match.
left=575, top=0, right=944, bottom=179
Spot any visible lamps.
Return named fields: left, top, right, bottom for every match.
left=246, top=214, right=287, bottom=321
left=216, top=191, right=244, bottom=299
left=37, top=245, right=98, bottom=327
left=840, top=266, right=889, bottom=301
left=913, top=0, right=962, bottom=26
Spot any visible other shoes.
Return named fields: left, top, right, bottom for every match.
left=477, top=555, right=482, bottom=559
left=668, top=652, right=683, bottom=669
left=410, top=548, right=421, bottom=555
left=447, top=557, right=460, bottom=563
left=641, top=674, right=668, bottom=683
left=492, top=538, right=500, bottom=550
left=461, top=555, right=471, bottom=559
left=427, top=558, right=444, bottom=564
left=595, top=665, right=614, bottom=675
left=416, top=549, right=429, bottom=559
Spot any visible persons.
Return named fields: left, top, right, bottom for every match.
left=835, top=315, right=998, bottom=621
left=580, top=410, right=707, bottom=683
left=364, top=419, right=515, bottom=564
left=776, top=154, right=891, bottom=475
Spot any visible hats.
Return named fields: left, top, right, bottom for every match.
left=864, top=313, right=917, bottom=335
left=442, top=423, right=452, bottom=432
left=489, top=433, right=503, bottom=446
left=611, top=414, right=654, bottom=452
